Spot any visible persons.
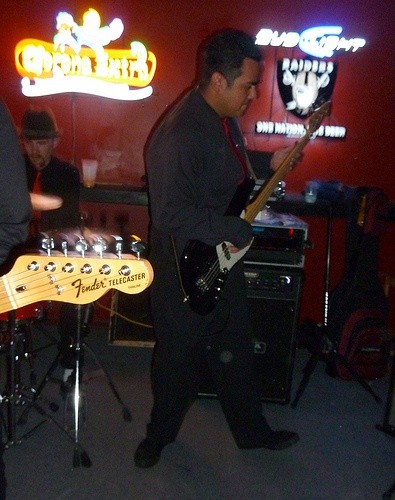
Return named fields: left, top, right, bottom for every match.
left=133, top=30, right=305, bottom=471
left=18, top=105, right=94, bottom=398
left=0, top=96, right=33, bottom=266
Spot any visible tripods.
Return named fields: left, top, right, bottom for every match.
left=0, top=304, right=133, bottom=469
left=290, top=204, right=382, bottom=408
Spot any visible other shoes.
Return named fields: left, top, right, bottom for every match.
left=133, top=429, right=179, bottom=468
left=241, top=429, right=301, bottom=452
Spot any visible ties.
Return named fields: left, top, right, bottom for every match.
left=28, top=171, right=44, bottom=240
left=220, top=118, right=249, bottom=188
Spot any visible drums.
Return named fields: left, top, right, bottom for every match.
left=1, top=246, right=59, bottom=325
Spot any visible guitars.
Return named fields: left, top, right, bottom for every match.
left=0, top=234, right=153, bottom=313
left=174, top=101, right=333, bottom=316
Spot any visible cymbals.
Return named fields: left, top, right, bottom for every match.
left=42, top=227, right=119, bottom=246
left=29, top=193, right=62, bottom=211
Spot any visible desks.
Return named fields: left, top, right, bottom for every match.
left=78, top=185, right=349, bottom=328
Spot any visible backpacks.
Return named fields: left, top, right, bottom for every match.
left=328, top=307, right=390, bottom=384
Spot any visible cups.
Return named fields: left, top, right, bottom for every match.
left=305, top=180, right=318, bottom=203
left=81, top=159, right=98, bottom=189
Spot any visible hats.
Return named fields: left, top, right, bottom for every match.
left=17, top=104, right=59, bottom=141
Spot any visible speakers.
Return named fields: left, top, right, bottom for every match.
left=193, top=262, right=303, bottom=403
